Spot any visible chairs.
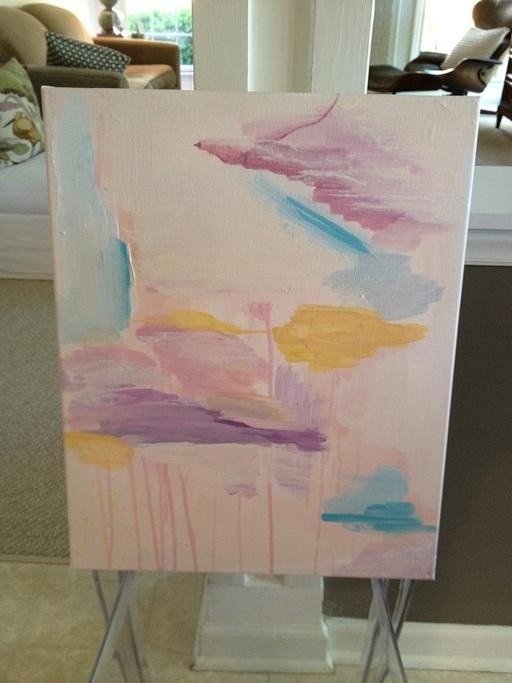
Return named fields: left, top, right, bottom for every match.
left=367, top=0, right=511, bottom=97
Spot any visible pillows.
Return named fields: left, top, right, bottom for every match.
left=439, top=25, right=510, bottom=70
left=44, top=30, right=132, bottom=78
left=0, top=57, right=45, bottom=167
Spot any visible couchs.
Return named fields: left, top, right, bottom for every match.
left=0, top=2, right=180, bottom=122
